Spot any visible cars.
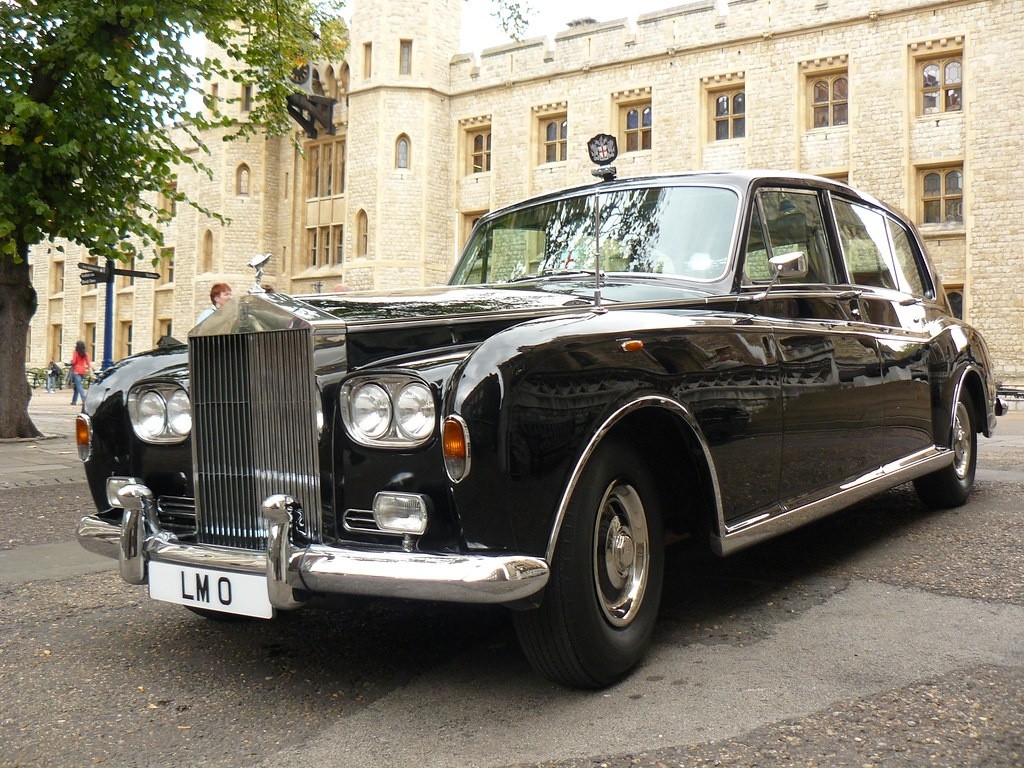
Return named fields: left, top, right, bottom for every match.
left=75, top=134, right=1013, bottom=693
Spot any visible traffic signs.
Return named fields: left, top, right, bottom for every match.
left=79, top=271, right=105, bottom=279
left=114, top=269, right=162, bottom=280
left=80, top=277, right=107, bottom=285
left=76, top=262, right=106, bottom=274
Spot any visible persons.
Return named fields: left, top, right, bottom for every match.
left=42, top=357, right=61, bottom=393
left=196, top=284, right=232, bottom=327
left=70, top=341, right=97, bottom=406
